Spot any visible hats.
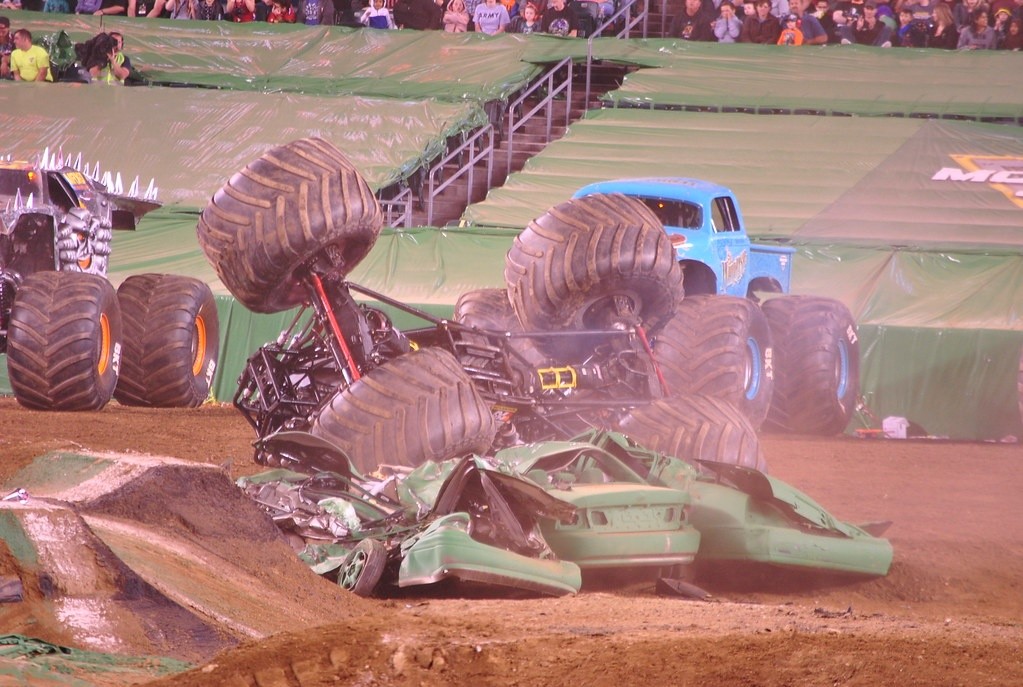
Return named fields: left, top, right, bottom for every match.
left=865, top=0, right=876, bottom=10
left=783, top=13, right=799, bottom=22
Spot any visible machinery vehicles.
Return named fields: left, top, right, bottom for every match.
left=567, top=175, right=862, bottom=433
left=0, top=143, right=221, bottom=413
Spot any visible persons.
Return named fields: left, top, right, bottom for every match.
left=89, top=32, right=132, bottom=85
left=0, top=0, right=1023, bottom=51
left=0, top=17, right=15, bottom=80
left=10, top=29, right=53, bottom=83
